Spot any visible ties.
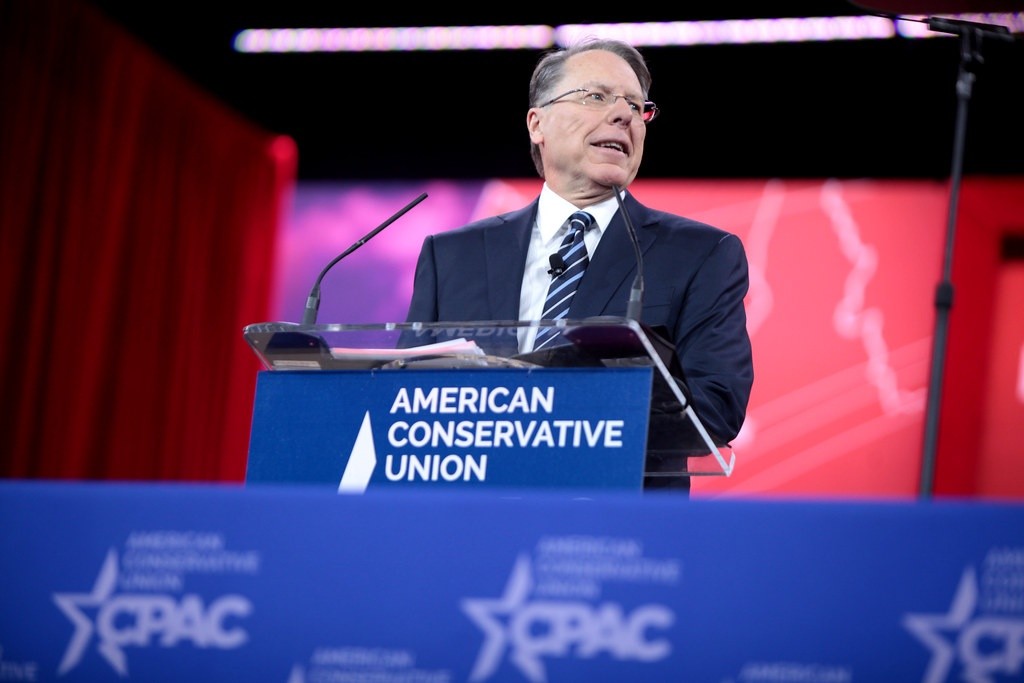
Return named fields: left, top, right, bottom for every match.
left=532, top=211, right=595, bottom=353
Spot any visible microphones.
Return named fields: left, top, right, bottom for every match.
left=563, top=185, right=676, bottom=368
left=928, top=17, right=1014, bottom=41
left=549, top=254, right=563, bottom=275
left=267, top=193, right=428, bottom=352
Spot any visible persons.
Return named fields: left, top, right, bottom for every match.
left=395, top=36, right=754, bottom=499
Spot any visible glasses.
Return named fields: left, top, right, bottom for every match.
left=539, top=87, right=660, bottom=127
